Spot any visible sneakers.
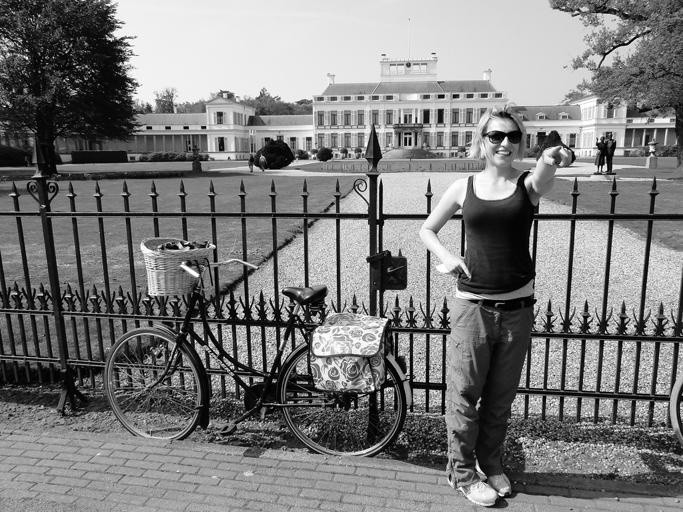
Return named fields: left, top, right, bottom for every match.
left=474, top=459, right=512, bottom=498
left=446, top=474, right=500, bottom=507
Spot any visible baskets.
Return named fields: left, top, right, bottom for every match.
left=140, top=236, right=218, bottom=297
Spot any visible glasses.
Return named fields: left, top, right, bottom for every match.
left=482, top=130, right=523, bottom=145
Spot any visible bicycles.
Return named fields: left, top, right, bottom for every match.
left=104, top=237, right=412, bottom=457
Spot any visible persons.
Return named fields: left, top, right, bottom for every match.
left=593, top=135, right=607, bottom=173
left=248, top=154, right=255, bottom=173
left=418, top=105, right=574, bottom=507
left=259, top=153, right=266, bottom=172
left=604, top=133, right=616, bottom=173
left=24, top=153, right=29, bottom=167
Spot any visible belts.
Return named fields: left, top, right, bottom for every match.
left=471, top=294, right=537, bottom=311
